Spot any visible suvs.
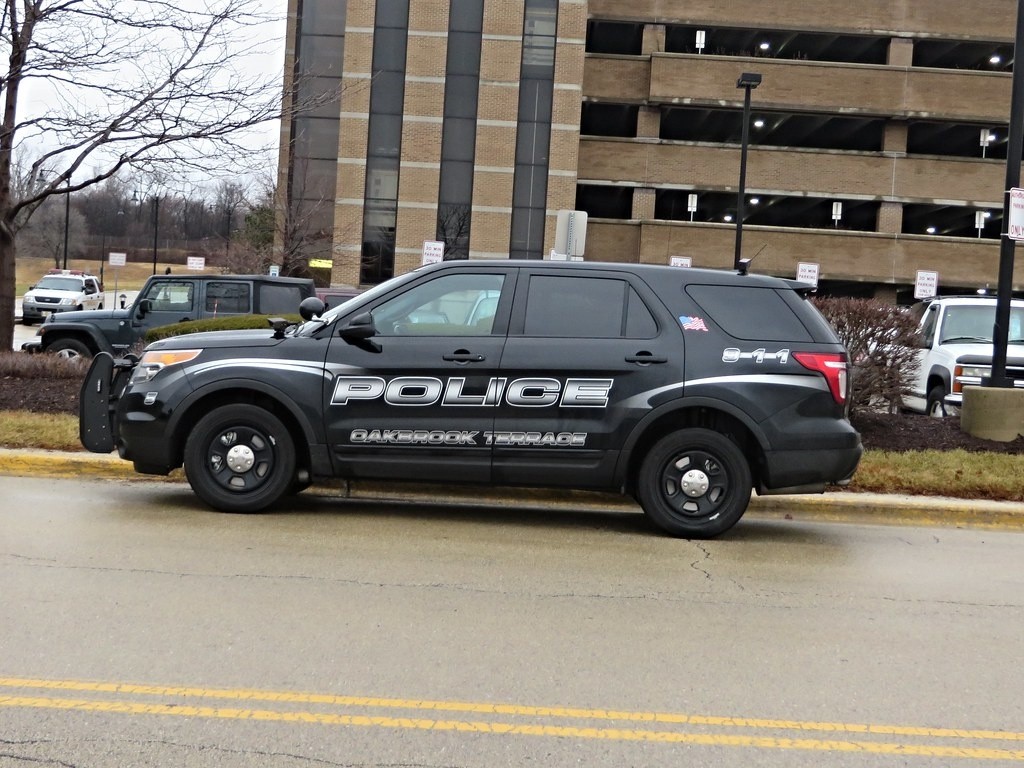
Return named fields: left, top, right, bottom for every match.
left=18, top=267, right=321, bottom=378
left=72, top=253, right=865, bottom=540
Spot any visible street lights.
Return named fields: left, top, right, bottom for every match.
left=732, top=73, right=763, bottom=271
left=35, top=169, right=72, bottom=269
left=100, top=205, right=125, bottom=285
left=206, top=204, right=231, bottom=275
left=131, top=189, right=159, bottom=275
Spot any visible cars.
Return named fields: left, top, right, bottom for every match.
left=839, top=293, right=1024, bottom=420
left=21, top=268, right=106, bottom=327
